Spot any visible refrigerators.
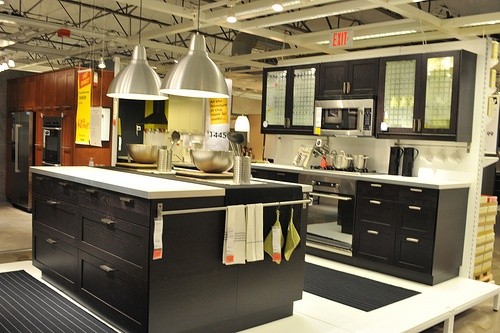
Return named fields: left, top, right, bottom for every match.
left=5, top=111, right=34, bottom=213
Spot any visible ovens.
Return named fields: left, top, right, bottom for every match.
left=297, top=174, right=357, bottom=258
left=41, top=116, right=62, bottom=167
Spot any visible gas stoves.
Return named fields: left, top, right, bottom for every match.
left=301, top=165, right=387, bottom=176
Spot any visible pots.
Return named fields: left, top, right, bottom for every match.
left=325, top=150, right=371, bottom=169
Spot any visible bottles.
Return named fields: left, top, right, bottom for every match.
left=88, top=157, right=95, bottom=166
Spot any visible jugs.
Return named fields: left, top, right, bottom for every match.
left=388, top=146, right=420, bottom=177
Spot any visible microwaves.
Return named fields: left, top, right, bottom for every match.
left=313, top=99, right=375, bottom=136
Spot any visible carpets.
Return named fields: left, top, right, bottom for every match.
left=303, top=260, right=423, bottom=313
left=0, top=267, right=118, bottom=333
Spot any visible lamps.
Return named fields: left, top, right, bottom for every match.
left=271, top=0, right=284, bottom=13
left=105, top=0, right=170, bottom=102
left=225, top=1, right=238, bottom=24
left=233, top=87, right=251, bottom=133
left=159, top=0, right=231, bottom=100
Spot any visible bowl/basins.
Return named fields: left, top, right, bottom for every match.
left=189, top=149, right=235, bottom=173
left=126, top=144, right=167, bottom=163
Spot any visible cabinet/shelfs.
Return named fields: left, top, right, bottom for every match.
left=30, top=173, right=313, bottom=333
left=375, top=48, right=478, bottom=145
left=4, top=64, right=113, bottom=214
left=319, top=57, right=379, bottom=101
left=351, top=181, right=394, bottom=277
left=394, top=188, right=470, bottom=288
left=260, top=62, right=318, bottom=136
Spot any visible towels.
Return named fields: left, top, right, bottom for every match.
left=223, top=205, right=247, bottom=265
left=246, top=203, right=265, bottom=263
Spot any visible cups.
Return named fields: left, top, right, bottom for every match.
left=156, top=149, right=172, bottom=172
